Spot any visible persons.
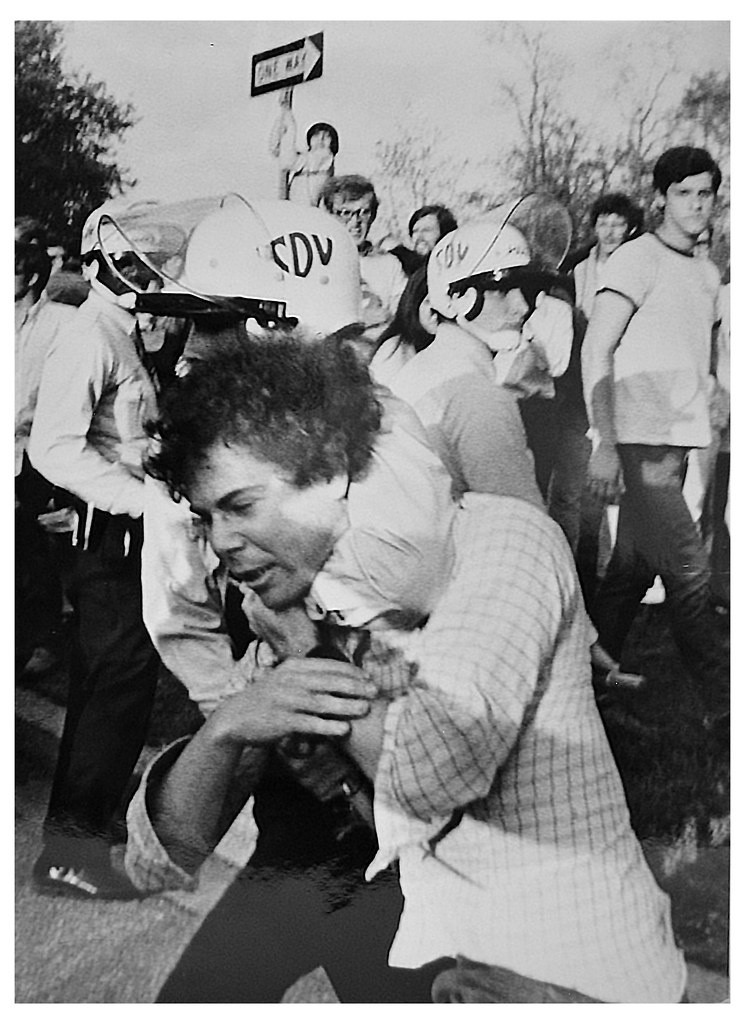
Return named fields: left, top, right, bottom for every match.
left=124, top=342, right=689, bottom=1005
left=97, top=194, right=454, bottom=1004
left=15, top=100, right=731, bottom=900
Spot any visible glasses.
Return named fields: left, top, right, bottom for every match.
left=333, top=207, right=371, bottom=217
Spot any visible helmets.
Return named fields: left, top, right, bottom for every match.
left=426, top=220, right=532, bottom=319
left=81, top=194, right=187, bottom=256
left=134, top=193, right=366, bottom=349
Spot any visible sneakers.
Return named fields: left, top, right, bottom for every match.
left=32, top=842, right=154, bottom=900
